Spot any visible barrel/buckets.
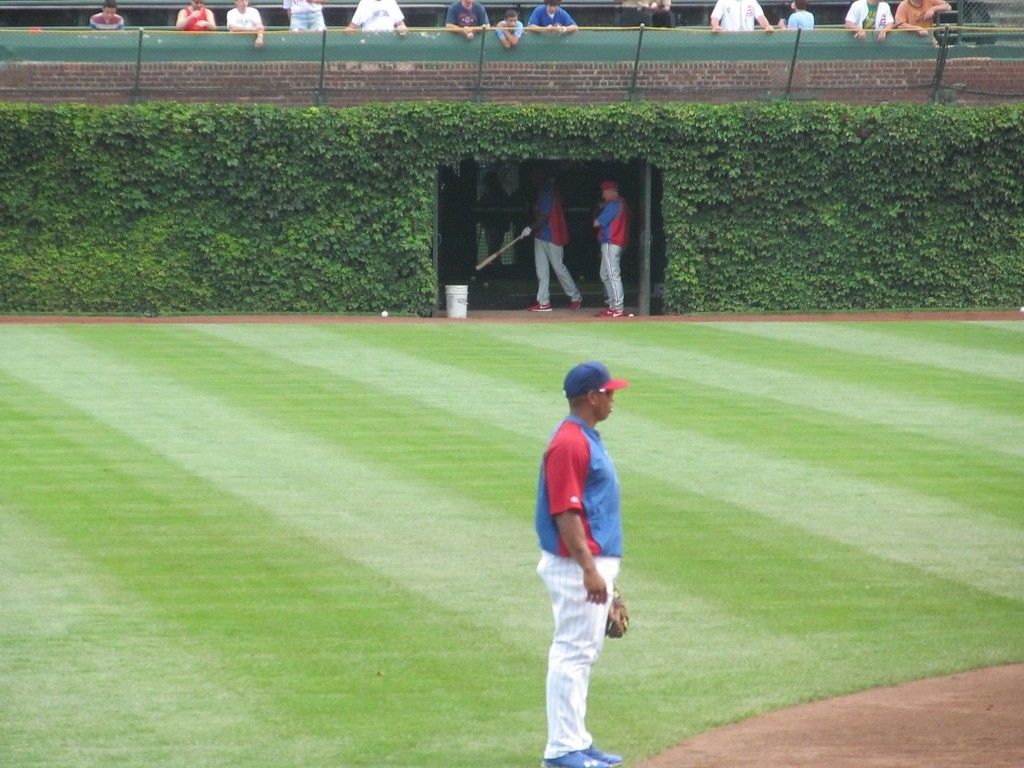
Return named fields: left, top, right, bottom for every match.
left=445, top=285, right=468, bottom=293
left=445, top=292, right=467, bottom=318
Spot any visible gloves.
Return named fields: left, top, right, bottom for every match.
left=520, top=226, right=533, bottom=240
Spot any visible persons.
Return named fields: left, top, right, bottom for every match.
left=283, top=0, right=328, bottom=31
left=777, top=0, right=815, bottom=31
left=445, top=0, right=491, bottom=41
left=637, top=0, right=676, bottom=28
left=495, top=9, right=523, bottom=50
left=535, top=360, right=630, bottom=768
left=592, top=180, right=629, bottom=318
left=527, top=0, right=578, bottom=34
left=710, top=0, right=776, bottom=35
left=342, top=0, right=408, bottom=35
left=227, top=0, right=265, bottom=48
left=176, top=0, right=217, bottom=31
left=90, top=0, right=124, bottom=31
left=520, top=173, right=583, bottom=313
left=844, top=0, right=951, bottom=47
left=477, top=170, right=537, bottom=268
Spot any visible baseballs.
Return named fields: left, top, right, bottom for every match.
left=628, top=313, right=634, bottom=318
left=381, top=311, right=388, bottom=317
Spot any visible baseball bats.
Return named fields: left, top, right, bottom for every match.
left=475, top=235, right=521, bottom=272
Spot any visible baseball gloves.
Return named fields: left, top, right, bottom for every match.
left=605, top=583, right=629, bottom=639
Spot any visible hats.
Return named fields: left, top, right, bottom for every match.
left=600, top=180, right=618, bottom=191
left=562, top=361, right=629, bottom=397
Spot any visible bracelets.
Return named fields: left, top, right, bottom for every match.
left=563, top=26, right=567, bottom=32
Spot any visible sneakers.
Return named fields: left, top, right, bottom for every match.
left=596, top=308, right=625, bottom=318
left=570, top=296, right=583, bottom=311
left=579, top=743, right=623, bottom=767
left=527, top=304, right=552, bottom=311
left=541, top=750, right=609, bottom=768
